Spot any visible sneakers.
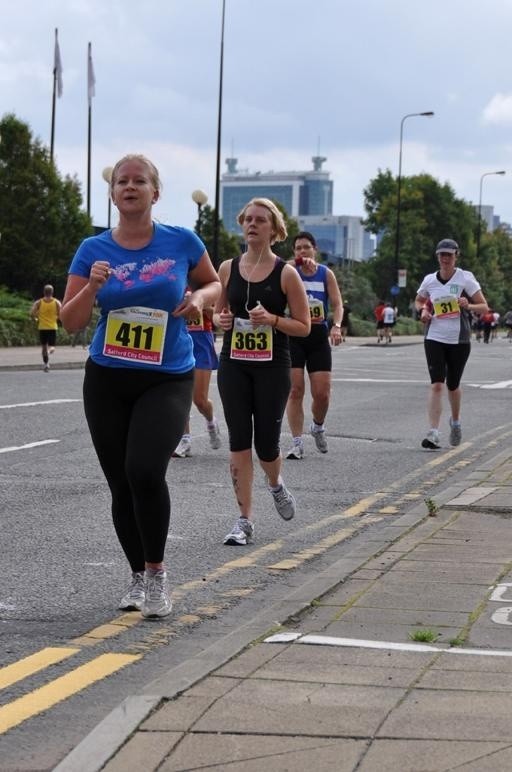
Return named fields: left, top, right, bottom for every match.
left=475, top=335, right=512, bottom=345
left=45, top=344, right=88, bottom=373
left=309, top=421, right=330, bottom=454
left=285, top=436, right=305, bottom=461
left=141, top=568, right=173, bottom=619
left=205, top=415, right=222, bottom=449
left=118, top=570, right=147, bottom=612
left=265, top=474, right=296, bottom=521
left=342, top=336, right=392, bottom=343
left=421, top=427, right=442, bottom=450
left=171, top=433, right=194, bottom=458
left=448, top=414, right=462, bottom=446
left=223, top=515, right=258, bottom=546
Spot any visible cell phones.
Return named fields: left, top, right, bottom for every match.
left=249, top=305, right=262, bottom=330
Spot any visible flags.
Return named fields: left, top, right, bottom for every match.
left=52, top=27, right=64, bottom=98
left=88, top=42, right=96, bottom=99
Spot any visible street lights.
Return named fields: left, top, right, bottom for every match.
left=99, top=163, right=117, bottom=231
left=476, top=168, right=506, bottom=258
left=393, top=109, right=435, bottom=311
left=191, top=187, right=209, bottom=239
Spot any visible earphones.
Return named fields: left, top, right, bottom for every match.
left=271, top=230, right=274, bottom=233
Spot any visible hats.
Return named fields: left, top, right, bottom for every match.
left=434, top=238, right=460, bottom=259
